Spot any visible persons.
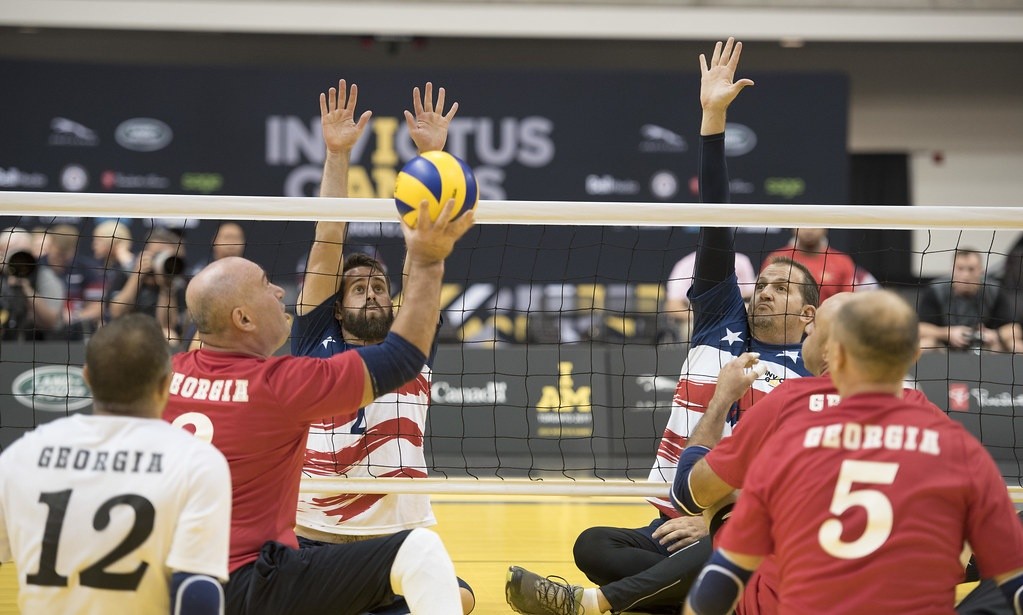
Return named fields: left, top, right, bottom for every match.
left=505, top=36, right=1023, bottom=615
left=0, top=79, right=476, bottom=615
left=0, top=220, right=1023, bottom=351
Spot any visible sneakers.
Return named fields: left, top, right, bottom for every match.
left=505, top=566, right=585, bottom=615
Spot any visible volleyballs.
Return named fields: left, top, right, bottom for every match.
left=394, top=151, right=480, bottom=229
